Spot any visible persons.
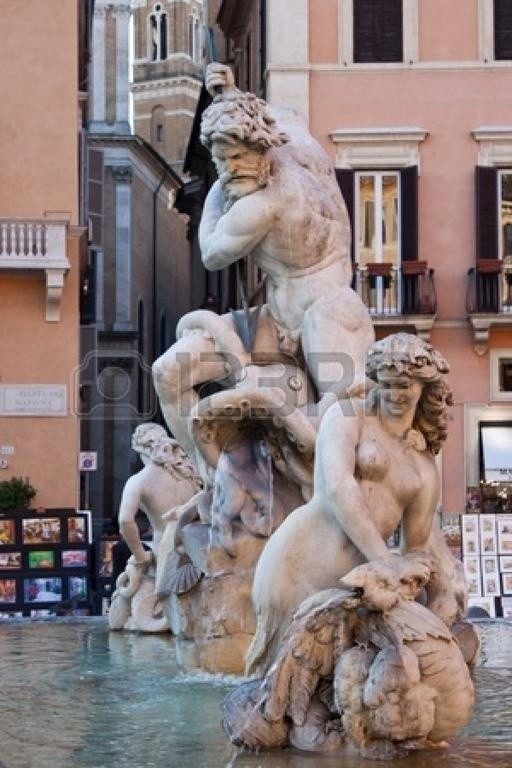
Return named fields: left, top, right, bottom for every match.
left=242, top=333, right=458, bottom=686
left=149, top=58, right=380, bottom=523
left=116, top=419, right=205, bottom=568
